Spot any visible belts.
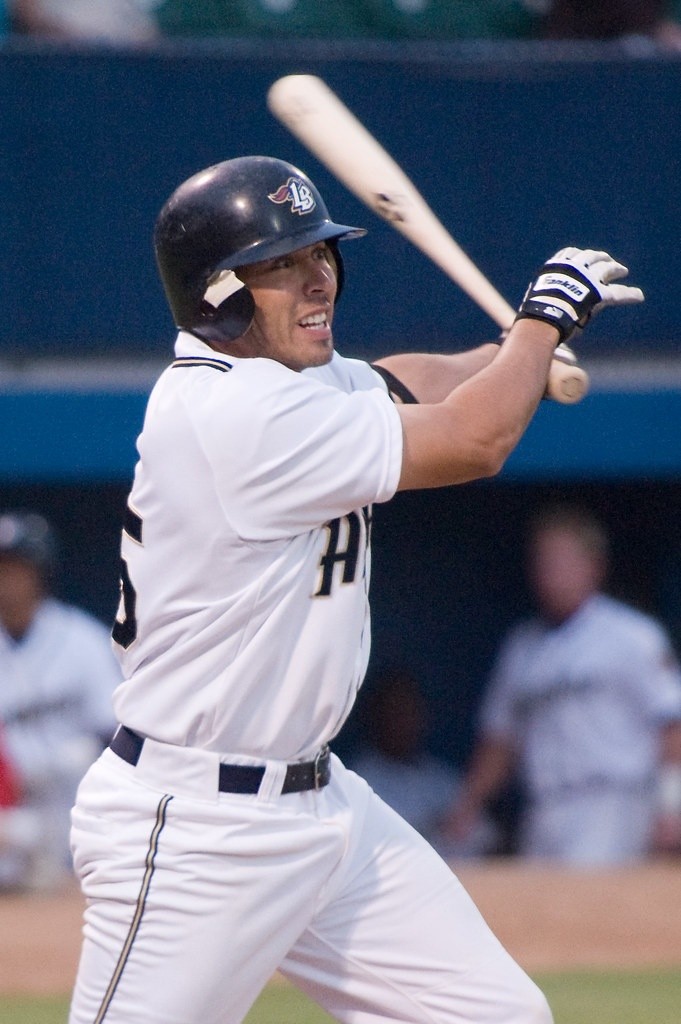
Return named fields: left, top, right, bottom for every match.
left=110, top=725, right=331, bottom=794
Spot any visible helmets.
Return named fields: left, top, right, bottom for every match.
left=153, top=155, right=368, bottom=341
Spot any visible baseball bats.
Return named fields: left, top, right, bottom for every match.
left=265, top=73, right=591, bottom=405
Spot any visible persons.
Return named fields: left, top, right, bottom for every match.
left=349, top=508, right=681, bottom=868
left=0, top=513, right=136, bottom=893
left=61, top=158, right=644, bottom=1024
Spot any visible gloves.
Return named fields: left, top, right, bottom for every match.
left=500, top=281, right=577, bottom=362
left=514, top=247, right=645, bottom=342
